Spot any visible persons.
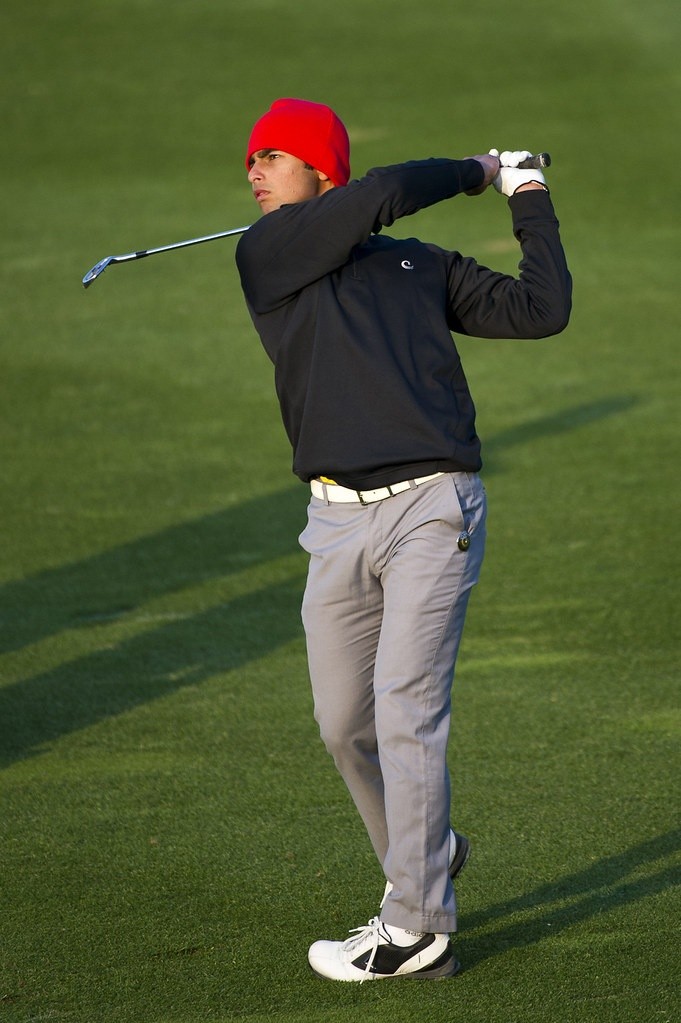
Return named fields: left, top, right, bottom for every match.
left=235, top=98, right=574, bottom=982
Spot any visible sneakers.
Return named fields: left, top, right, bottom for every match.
left=306, top=914, right=463, bottom=982
left=378, top=828, right=470, bottom=913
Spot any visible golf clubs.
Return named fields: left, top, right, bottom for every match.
left=80, top=151, right=553, bottom=291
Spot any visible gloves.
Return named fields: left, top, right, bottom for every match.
left=488, top=149, right=553, bottom=201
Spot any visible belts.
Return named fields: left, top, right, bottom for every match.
left=311, top=470, right=449, bottom=512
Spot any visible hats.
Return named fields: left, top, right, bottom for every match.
left=246, top=98, right=349, bottom=191
